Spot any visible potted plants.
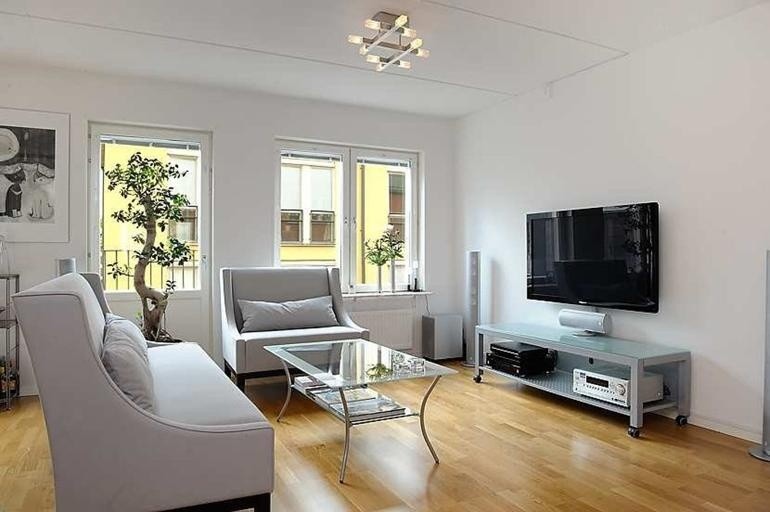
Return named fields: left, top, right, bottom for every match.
left=379, top=229, right=406, bottom=294
left=362, top=238, right=397, bottom=294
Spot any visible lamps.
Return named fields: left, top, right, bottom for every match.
left=344, top=10, right=432, bottom=74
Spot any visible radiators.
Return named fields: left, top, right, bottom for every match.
left=349, top=309, right=415, bottom=350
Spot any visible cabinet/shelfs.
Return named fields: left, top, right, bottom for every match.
left=469, top=319, right=694, bottom=438
left=0, top=274, right=25, bottom=412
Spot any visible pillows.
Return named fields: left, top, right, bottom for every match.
left=101, top=310, right=157, bottom=417
left=237, top=295, right=339, bottom=333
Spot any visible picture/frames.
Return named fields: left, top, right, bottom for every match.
left=1, top=106, right=73, bottom=245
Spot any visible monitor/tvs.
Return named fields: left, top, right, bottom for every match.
left=526, top=202, right=659, bottom=313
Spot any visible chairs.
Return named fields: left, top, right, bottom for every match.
left=217, top=263, right=373, bottom=395
left=10, top=272, right=278, bottom=512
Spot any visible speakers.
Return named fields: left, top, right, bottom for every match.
left=560, top=309, right=612, bottom=335
left=460, top=250, right=481, bottom=367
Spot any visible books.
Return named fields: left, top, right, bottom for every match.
left=293, top=372, right=406, bottom=421
